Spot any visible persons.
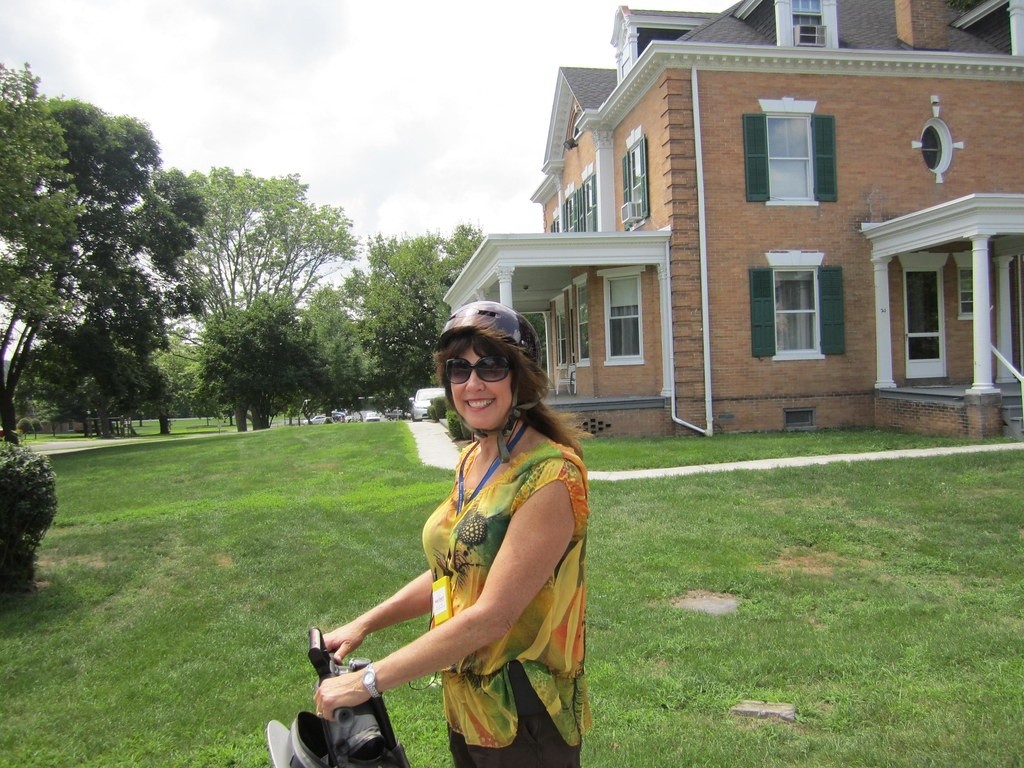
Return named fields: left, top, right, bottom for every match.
left=312, top=302, right=590, bottom=768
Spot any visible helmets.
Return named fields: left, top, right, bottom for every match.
left=440, top=301, right=542, bottom=369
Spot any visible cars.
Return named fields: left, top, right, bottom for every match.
left=385, top=409, right=412, bottom=421
left=408, top=387, right=446, bottom=422
left=308, top=410, right=380, bottom=424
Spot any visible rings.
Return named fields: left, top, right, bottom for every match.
left=317, top=712, right=323, bottom=716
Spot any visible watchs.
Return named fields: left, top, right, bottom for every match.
left=362, top=663, right=382, bottom=697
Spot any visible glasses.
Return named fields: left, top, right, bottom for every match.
left=445, top=355, right=514, bottom=384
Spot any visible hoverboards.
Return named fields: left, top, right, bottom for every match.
left=263, top=627, right=414, bottom=768
